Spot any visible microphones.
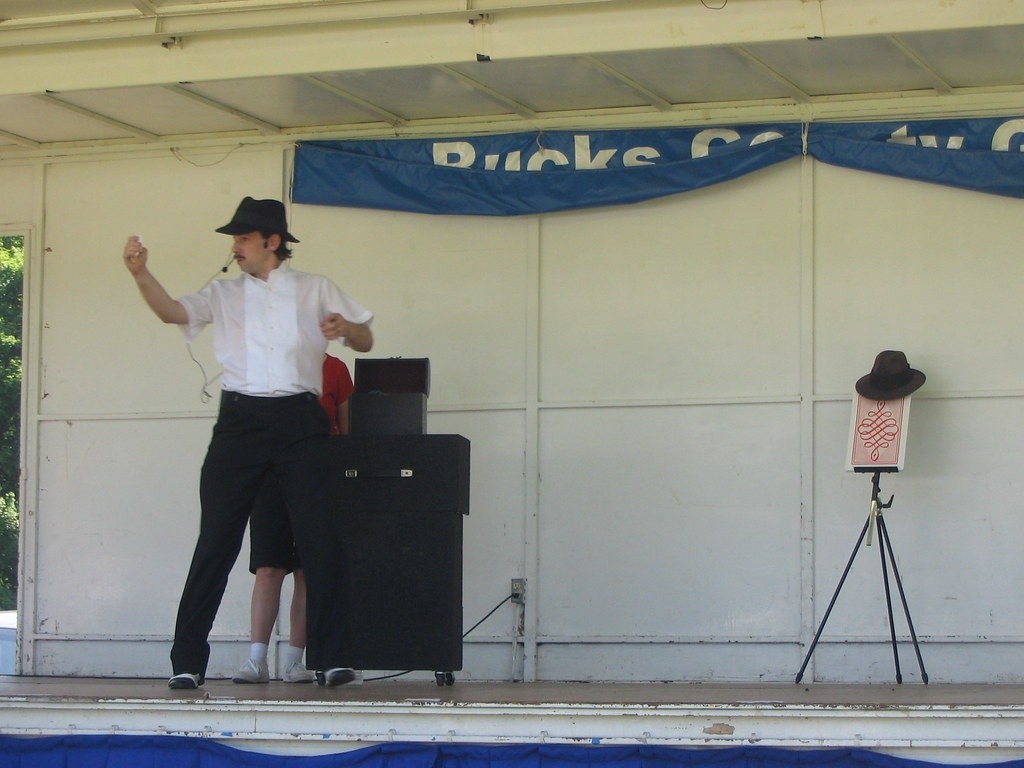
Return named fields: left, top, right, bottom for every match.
left=222, top=256, right=235, bottom=273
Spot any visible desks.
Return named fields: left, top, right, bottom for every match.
left=302, top=434, right=471, bottom=688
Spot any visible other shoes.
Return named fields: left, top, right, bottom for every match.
left=167, top=673, right=205, bottom=689
left=316, top=667, right=356, bottom=687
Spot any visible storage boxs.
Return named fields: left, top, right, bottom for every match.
left=348, top=391, right=427, bottom=433
left=354, top=357, right=431, bottom=394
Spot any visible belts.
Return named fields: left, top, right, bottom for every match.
left=222, top=390, right=317, bottom=407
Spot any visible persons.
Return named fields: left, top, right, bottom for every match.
left=232, top=351, right=354, bottom=683
left=122, top=197, right=375, bottom=687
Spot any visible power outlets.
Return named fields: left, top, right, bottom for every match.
left=511, top=578, right=526, bottom=602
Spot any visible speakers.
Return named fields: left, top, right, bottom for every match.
left=306, top=512, right=463, bottom=671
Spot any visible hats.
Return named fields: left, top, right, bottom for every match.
left=855, top=350, right=926, bottom=400
left=215, top=196, right=300, bottom=244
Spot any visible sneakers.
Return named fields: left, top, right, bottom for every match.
left=283, top=662, right=314, bottom=683
left=232, top=658, right=269, bottom=684
